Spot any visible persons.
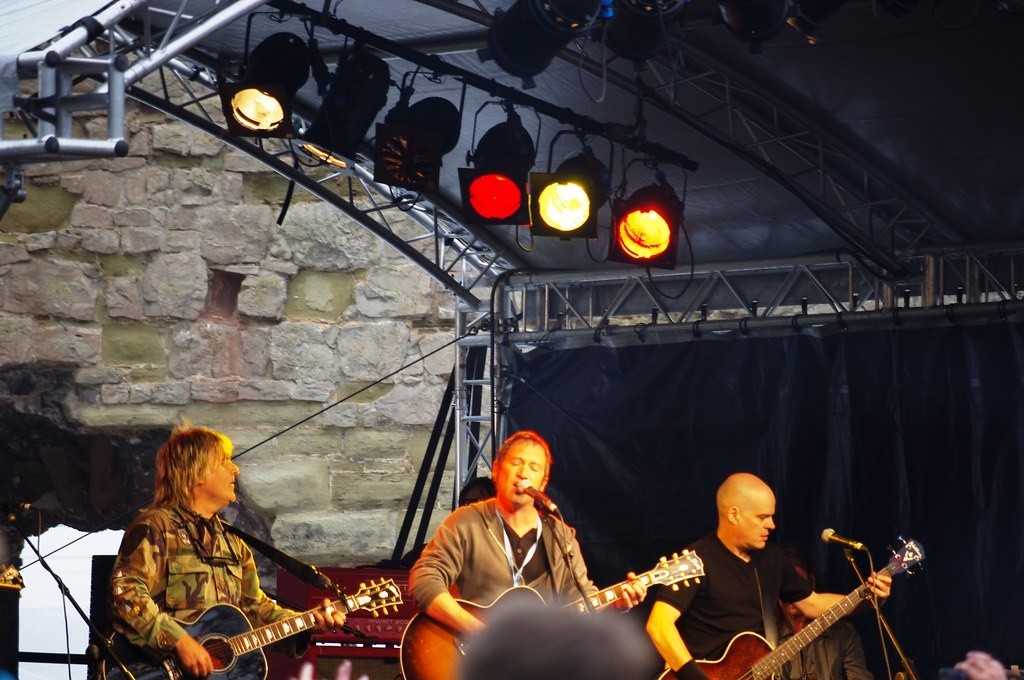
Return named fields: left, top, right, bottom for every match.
left=643, top=472, right=893, bottom=680
left=104, top=426, right=347, bottom=678
left=406, top=429, right=648, bottom=643
left=953, top=651, right=1006, bottom=680
left=773, top=555, right=874, bottom=680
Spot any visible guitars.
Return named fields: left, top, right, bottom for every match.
left=399, top=549, right=706, bottom=680
left=100, top=577, right=404, bottom=680
left=657, top=533, right=927, bottom=680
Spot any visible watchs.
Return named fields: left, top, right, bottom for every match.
left=616, top=608, right=631, bottom=616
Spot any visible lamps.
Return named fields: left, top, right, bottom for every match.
left=232, top=30, right=309, bottom=133
left=303, top=49, right=391, bottom=169
left=607, top=186, right=682, bottom=270
left=591, top=0, right=685, bottom=61
left=531, top=153, right=611, bottom=237
left=477, top=0, right=603, bottom=90
left=458, top=122, right=535, bottom=225
left=711, top=0, right=798, bottom=54
left=370, top=96, right=461, bottom=192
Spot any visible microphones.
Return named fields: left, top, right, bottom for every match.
left=0, top=497, right=37, bottom=511
left=821, top=529, right=868, bottom=552
left=517, top=480, right=558, bottom=512
